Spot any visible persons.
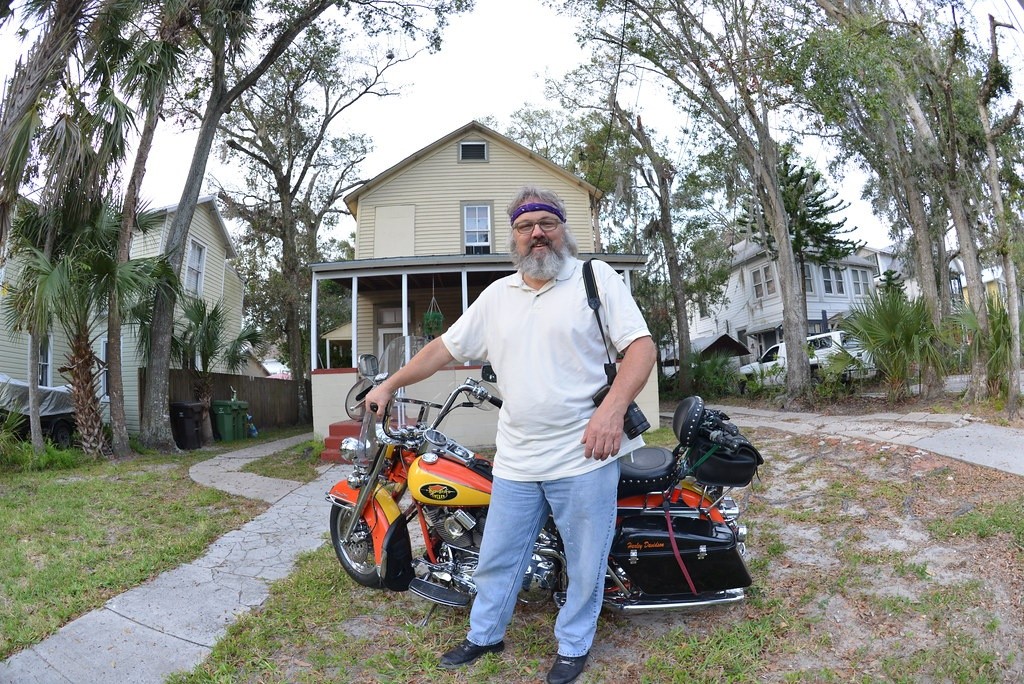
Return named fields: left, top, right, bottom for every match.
left=365, top=186, right=657, bottom=684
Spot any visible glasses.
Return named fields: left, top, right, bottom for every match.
left=513, top=217, right=564, bottom=234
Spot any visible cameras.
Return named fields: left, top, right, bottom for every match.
left=592, top=383, right=651, bottom=440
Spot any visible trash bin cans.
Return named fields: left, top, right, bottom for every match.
left=232, top=401, right=249, bottom=442
left=211, top=399, right=239, bottom=443
left=169, top=400, right=204, bottom=450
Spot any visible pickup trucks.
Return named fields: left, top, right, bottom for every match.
left=739, top=328, right=878, bottom=398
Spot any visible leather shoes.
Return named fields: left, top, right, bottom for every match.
left=440, top=638, right=505, bottom=667
left=544, top=650, right=590, bottom=684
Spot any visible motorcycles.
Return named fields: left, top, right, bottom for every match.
left=323, top=334, right=764, bottom=619
